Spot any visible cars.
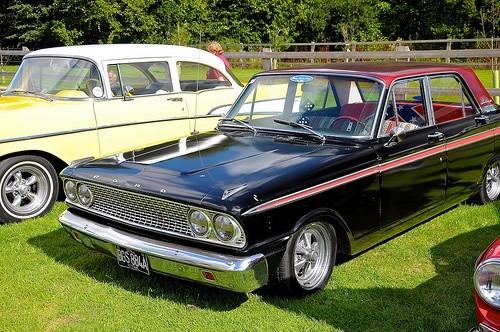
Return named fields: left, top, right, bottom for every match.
left=0, top=45, right=245, bottom=224
left=468, top=236, right=500, bottom=332
left=59, top=62, right=500, bottom=299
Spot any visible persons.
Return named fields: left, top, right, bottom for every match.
left=206, top=41, right=230, bottom=82
left=107, top=70, right=126, bottom=96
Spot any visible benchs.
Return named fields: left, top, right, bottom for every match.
left=144, top=83, right=217, bottom=93
left=303, top=117, right=418, bottom=136
left=342, top=102, right=472, bottom=124
